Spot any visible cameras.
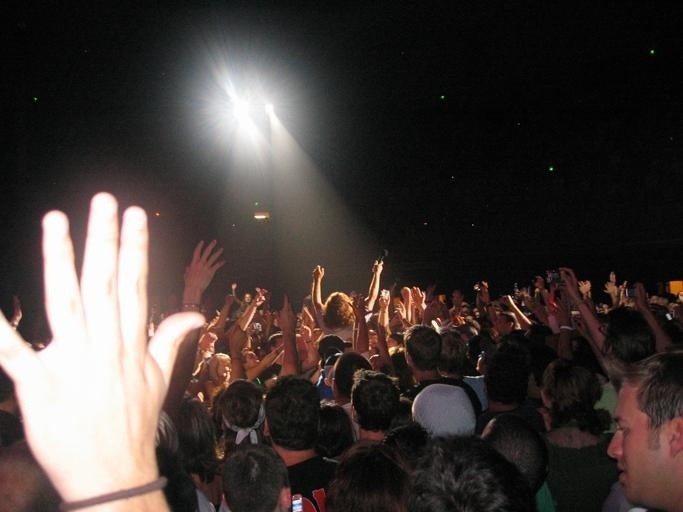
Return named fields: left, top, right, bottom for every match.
left=546, top=269, right=565, bottom=284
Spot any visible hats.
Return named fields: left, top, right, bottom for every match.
left=317, top=335, right=354, bottom=358
left=411, top=381, right=477, bottom=439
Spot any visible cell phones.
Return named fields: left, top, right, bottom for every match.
left=625, top=288, right=637, bottom=297
left=291, top=493, right=303, bottom=512
